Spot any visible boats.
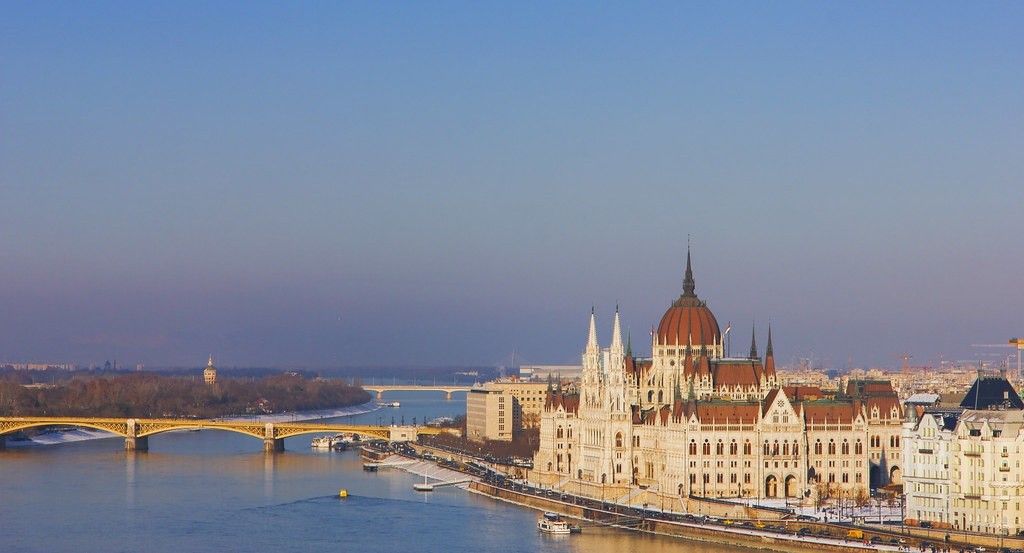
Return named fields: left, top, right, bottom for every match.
left=536, top=511, right=584, bottom=537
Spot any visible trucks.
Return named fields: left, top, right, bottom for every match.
left=847, top=530, right=863, bottom=539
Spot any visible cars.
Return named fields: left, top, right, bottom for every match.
left=560, top=493, right=594, bottom=506
left=459, top=467, right=501, bottom=486
left=920, top=521, right=934, bottom=528
left=535, top=490, right=542, bottom=494
left=644, top=511, right=669, bottom=519
left=604, top=505, right=623, bottom=513
left=547, top=489, right=554, bottom=497
left=700, top=515, right=786, bottom=531
left=685, top=513, right=694, bottom=519
left=891, top=537, right=907, bottom=544
left=871, top=536, right=882, bottom=540
left=976, top=546, right=986, bottom=552
left=1016, top=531, right=1024, bottom=536
left=503, top=480, right=509, bottom=486
left=818, top=529, right=831, bottom=537
left=797, top=527, right=811, bottom=534
left=391, top=442, right=445, bottom=464
left=523, top=486, right=529, bottom=491
